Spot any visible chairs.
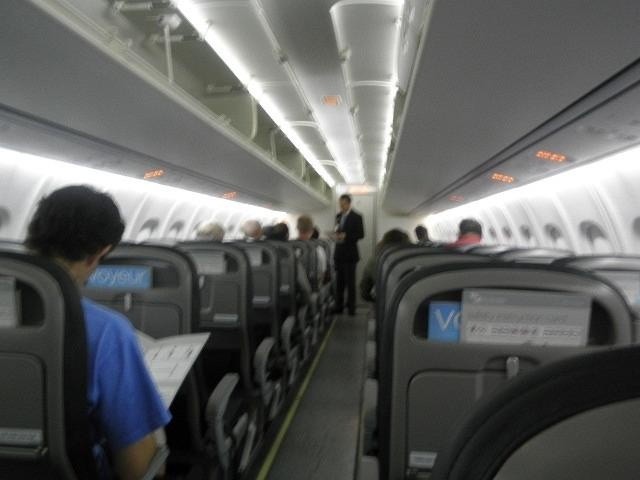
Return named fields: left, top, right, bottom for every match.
left=358, top=239, right=640, bottom=479
left=0, top=236, right=333, bottom=479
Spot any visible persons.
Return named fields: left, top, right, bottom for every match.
left=21, top=184, right=173, bottom=479
left=361, top=219, right=482, bottom=301
left=329, top=194, right=364, bottom=315
left=198, top=216, right=328, bottom=288
